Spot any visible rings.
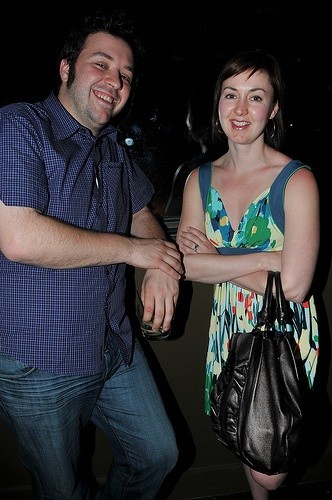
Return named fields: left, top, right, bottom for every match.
left=194, top=243, right=199, bottom=253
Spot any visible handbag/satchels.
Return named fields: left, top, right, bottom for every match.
left=209, top=269, right=321, bottom=476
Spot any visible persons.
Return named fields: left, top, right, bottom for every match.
left=165, top=94, right=229, bottom=217
left=0, top=11, right=185, bottom=500
left=174, top=50, right=321, bottom=500
